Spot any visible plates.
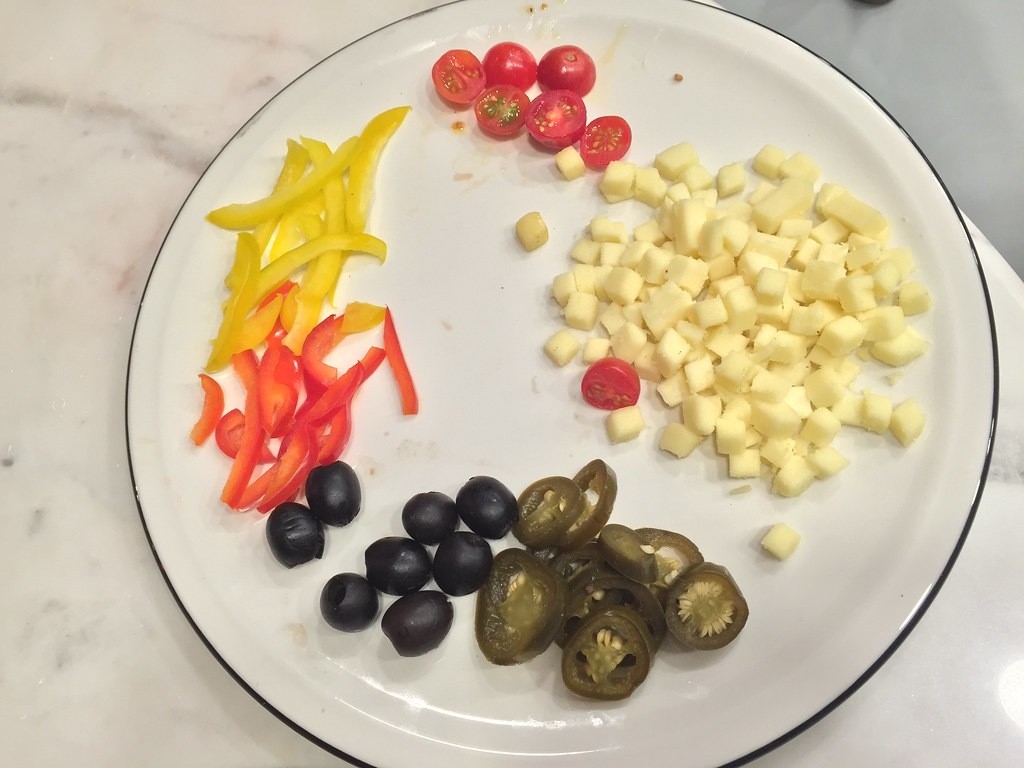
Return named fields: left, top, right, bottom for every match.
left=122, top=0, right=999, bottom=767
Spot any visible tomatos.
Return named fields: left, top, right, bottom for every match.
left=432, top=41, right=632, bottom=168
left=579, top=358, right=642, bottom=410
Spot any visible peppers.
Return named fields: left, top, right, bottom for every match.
left=473, top=459, right=748, bottom=700
left=192, top=106, right=418, bottom=516
